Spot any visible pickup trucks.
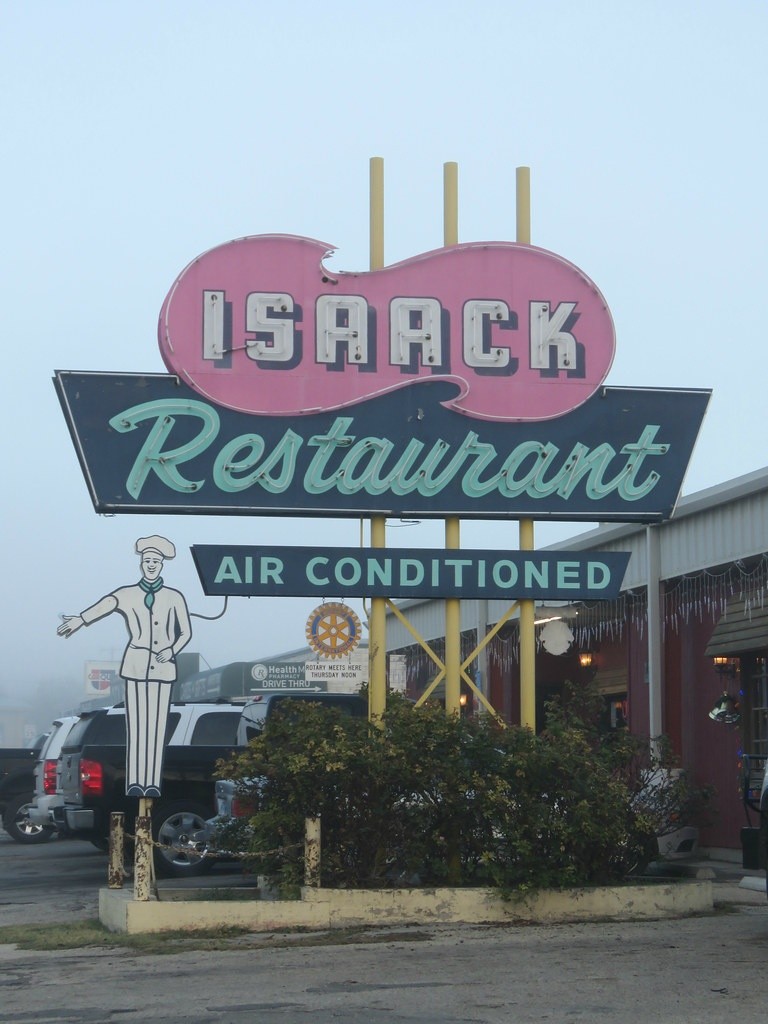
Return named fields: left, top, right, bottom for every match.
left=0, top=748, right=53, bottom=844
left=47, top=692, right=369, bottom=880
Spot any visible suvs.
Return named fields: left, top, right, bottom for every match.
left=54, top=700, right=242, bottom=784
left=26, top=716, right=81, bottom=831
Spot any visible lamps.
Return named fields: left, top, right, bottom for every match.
left=712, top=657, right=737, bottom=683
left=577, top=646, right=596, bottom=676
left=460, top=689, right=469, bottom=715
